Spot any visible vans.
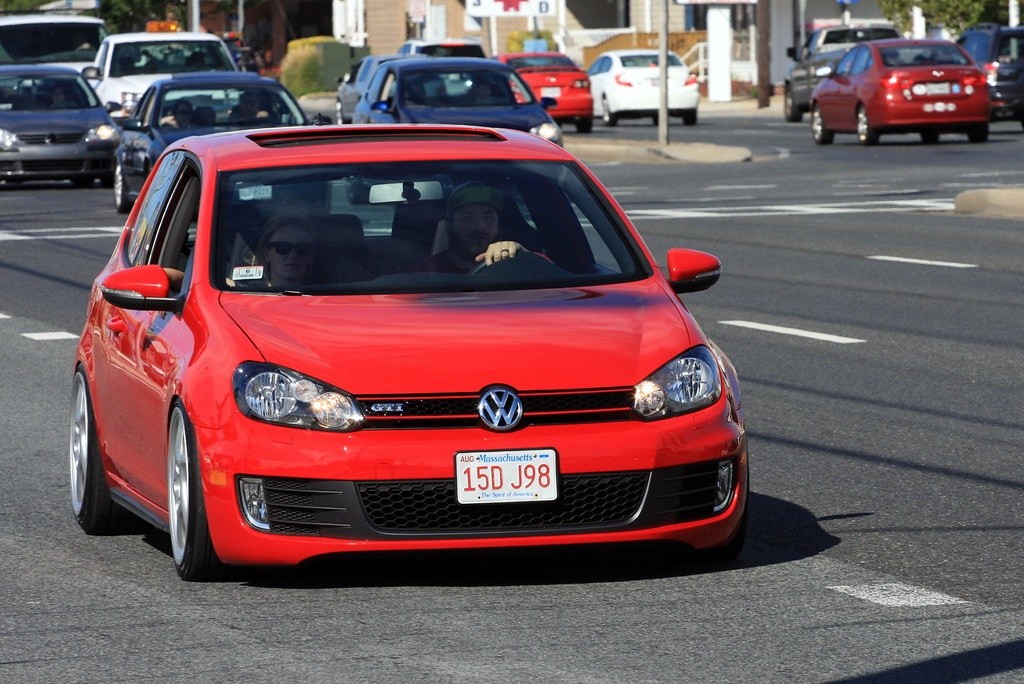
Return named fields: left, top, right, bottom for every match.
left=389, top=39, right=493, bottom=96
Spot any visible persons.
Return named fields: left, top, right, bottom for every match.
left=48, top=87, right=70, bottom=107
left=432, top=181, right=555, bottom=280
left=255, top=206, right=322, bottom=288
left=229, top=93, right=268, bottom=125
left=471, top=81, right=501, bottom=105
left=160, top=98, right=200, bottom=130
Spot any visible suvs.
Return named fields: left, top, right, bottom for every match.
left=949, top=23, right=1023, bottom=123
left=0, top=15, right=109, bottom=90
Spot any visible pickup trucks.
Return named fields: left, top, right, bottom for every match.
left=783, top=22, right=905, bottom=125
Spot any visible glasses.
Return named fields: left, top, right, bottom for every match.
left=267, top=240, right=312, bottom=256
left=178, top=109, right=192, bottom=115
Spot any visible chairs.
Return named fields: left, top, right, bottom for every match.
left=392, top=202, right=542, bottom=273
left=229, top=215, right=371, bottom=286
left=192, top=107, right=216, bottom=125
left=6, top=94, right=29, bottom=110
left=120, top=57, right=134, bottom=72
left=408, top=74, right=446, bottom=101
left=186, top=52, right=204, bottom=67
left=228, top=105, right=257, bottom=122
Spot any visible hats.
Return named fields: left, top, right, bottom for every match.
left=448, top=184, right=505, bottom=212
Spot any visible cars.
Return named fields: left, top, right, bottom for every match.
left=111, top=73, right=336, bottom=215
left=64, top=125, right=749, bottom=586
left=1, top=64, right=123, bottom=190
left=589, top=48, right=700, bottom=128
left=807, top=38, right=992, bottom=145
left=350, top=57, right=564, bottom=150
left=82, top=32, right=248, bottom=143
left=335, top=52, right=448, bottom=124
left=466, top=53, right=594, bottom=133
